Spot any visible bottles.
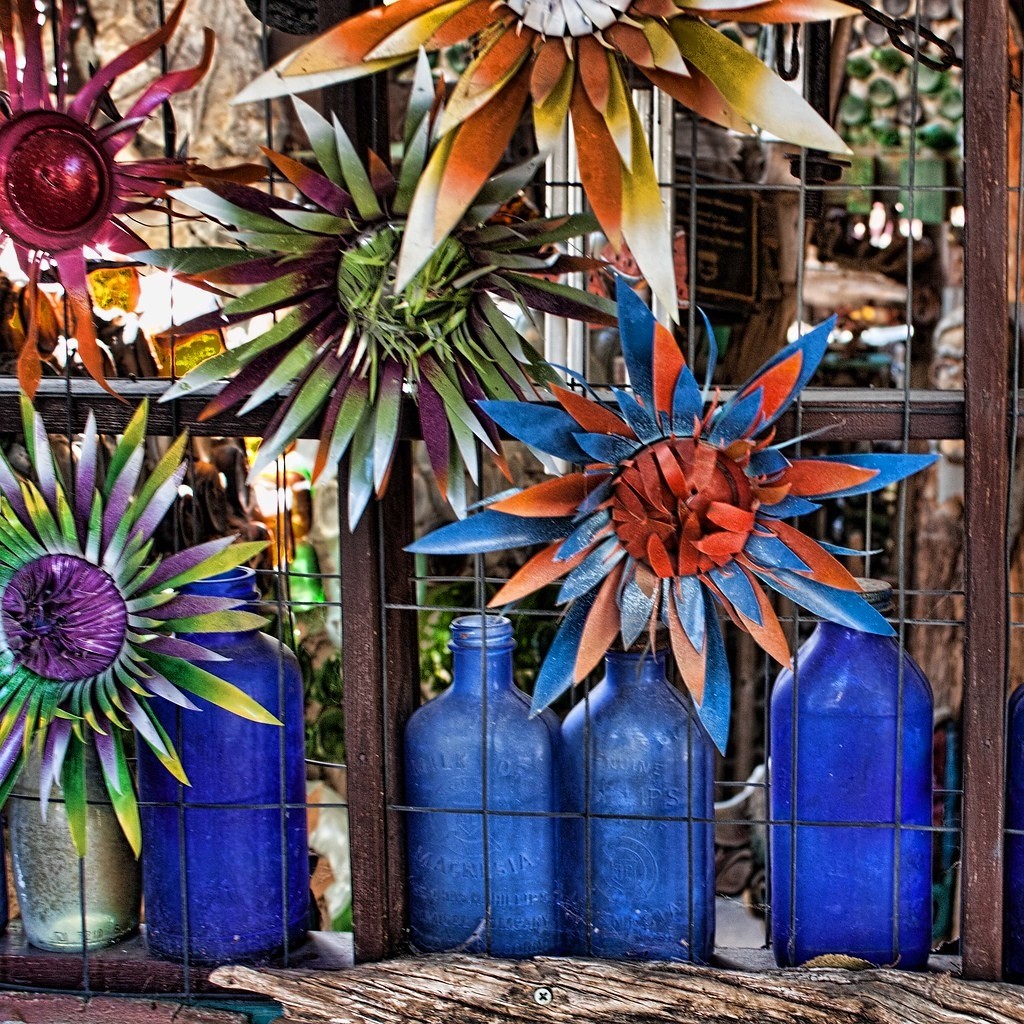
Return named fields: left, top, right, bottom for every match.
left=763, top=609, right=939, bottom=975
left=551, top=639, right=719, bottom=964
left=11, top=729, right=139, bottom=954
left=140, top=559, right=310, bottom=966
left=406, top=611, right=564, bottom=953
